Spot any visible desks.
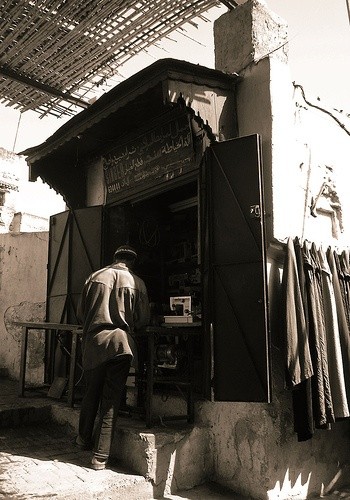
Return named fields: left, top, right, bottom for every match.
left=11, top=320, right=83, bottom=407
left=73, top=325, right=198, bottom=428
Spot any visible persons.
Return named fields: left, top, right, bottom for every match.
left=72, top=243, right=151, bottom=472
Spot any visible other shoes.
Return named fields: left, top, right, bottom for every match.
left=74, top=432, right=88, bottom=449
left=91, top=455, right=108, bottom=470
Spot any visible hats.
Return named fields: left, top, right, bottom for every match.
left=114, top=244, right=138, bottom=258
